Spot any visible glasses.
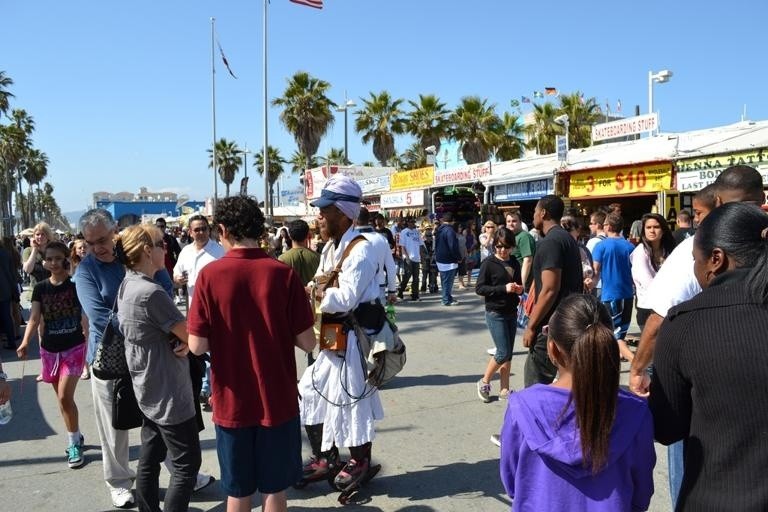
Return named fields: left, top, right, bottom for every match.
left=192, top=227, right=208, bottom=232
left=542, top=325, right=549, bottom=335
left=495, top=244, right=512, bottom=250
left=155, top=240, right=165, bottom=249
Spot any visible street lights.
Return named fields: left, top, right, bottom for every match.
left=647, top=68, right=671, bottom=137
left=332, top=89, right=356, bottom=167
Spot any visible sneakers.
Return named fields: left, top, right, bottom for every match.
left=0, top=400, right=13, bottom=425
left=487, top=347, right=497, bottom=356
left=193, top=473, right=216, bottom=492
left=491, top=434, right=501, bottom=446
left=301, top=449, right=341, bottom=481
left=441, top=299, right=459, bottom=306
left=65, top=434, right=85, bottom=468
left=477, top=378, right=516, bottom=403
left=111, top=486, right=135, bottom=508
left=335, top=457, right=370, bottom=490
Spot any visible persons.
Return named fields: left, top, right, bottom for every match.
left=381, top=211, right=478, bottom=307
left=474, top=163, right=768, bottom=510
left=0, top=166, right=398, bottom=512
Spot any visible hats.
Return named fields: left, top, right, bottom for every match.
left=310, top=190, right=362, bottom=208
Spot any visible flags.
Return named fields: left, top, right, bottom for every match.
left=616, top=98, right=621, bottom=112
left=591, top=98, right=596, bottom=111
left=522, top=95, right=531, bottom=103
left=545, top=88, right=556, bottom=95
left=291, top=0, right=323, bottom=8
left=214, top=30, right=237, bottom=79
left=606, top=98, right=611, bottom=112
left=580, top=89, right=584, bottom=102
left=511, top=99, right=519, bottom=106
left=533, top=91, right=543, bottom=97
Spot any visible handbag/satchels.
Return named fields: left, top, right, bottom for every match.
left=92, top=317, right=129, bottom=380
left=354, top=320, right=407, bottom=386
left=111, top=378, right=143, bottom=430
